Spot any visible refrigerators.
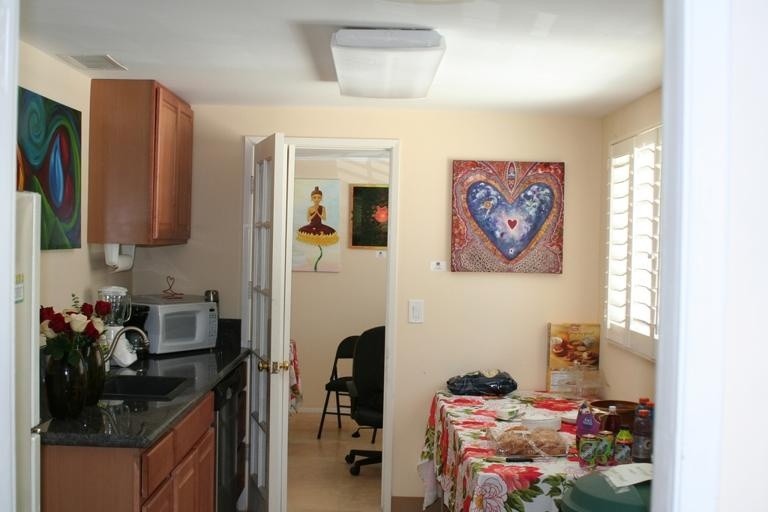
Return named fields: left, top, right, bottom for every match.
left=14, top=190, right=44, bottom=512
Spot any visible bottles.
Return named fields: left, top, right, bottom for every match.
left=630, top=396, right=655, bottom=462
left=604, top=406, right=622, bottom=435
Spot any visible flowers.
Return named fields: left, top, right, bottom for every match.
left=40, top=292, right=113, bottom=368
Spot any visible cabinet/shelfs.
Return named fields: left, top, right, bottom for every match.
left=40, top=391, right=215, bottom=512
left=88, top=78, right=192, bottom=247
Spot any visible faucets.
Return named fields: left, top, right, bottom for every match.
left=103, top=326, right=150, bottom=362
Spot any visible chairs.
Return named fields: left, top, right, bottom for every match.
left=317, top=325, right=384, bottom=475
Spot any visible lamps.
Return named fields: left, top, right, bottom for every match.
left=330, top=27, right=446, bottom=98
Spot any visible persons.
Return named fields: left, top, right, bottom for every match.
left=297, top=187, right=338, bottom=249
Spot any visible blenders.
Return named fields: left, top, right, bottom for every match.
left=93, top=285, right=140, bottom=368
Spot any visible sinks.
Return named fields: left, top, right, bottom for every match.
left=101, top=375, right=188, bottom=401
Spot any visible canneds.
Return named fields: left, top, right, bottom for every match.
left=579, top=433, right=600, bottom=470
left=598, top=430, right=616, bottom=466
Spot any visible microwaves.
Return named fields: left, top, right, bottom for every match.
left=145, top=353, right=217, bottom=409
left=129, top=293, right=219, bottom=354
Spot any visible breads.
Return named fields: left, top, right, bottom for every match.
left=497, top=423, right=567, bottom=459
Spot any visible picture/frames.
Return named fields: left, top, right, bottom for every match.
left=346, top=182, right=389, bottom=251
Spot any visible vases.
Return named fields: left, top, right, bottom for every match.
left=46, top=343, right=106, bottom=419
